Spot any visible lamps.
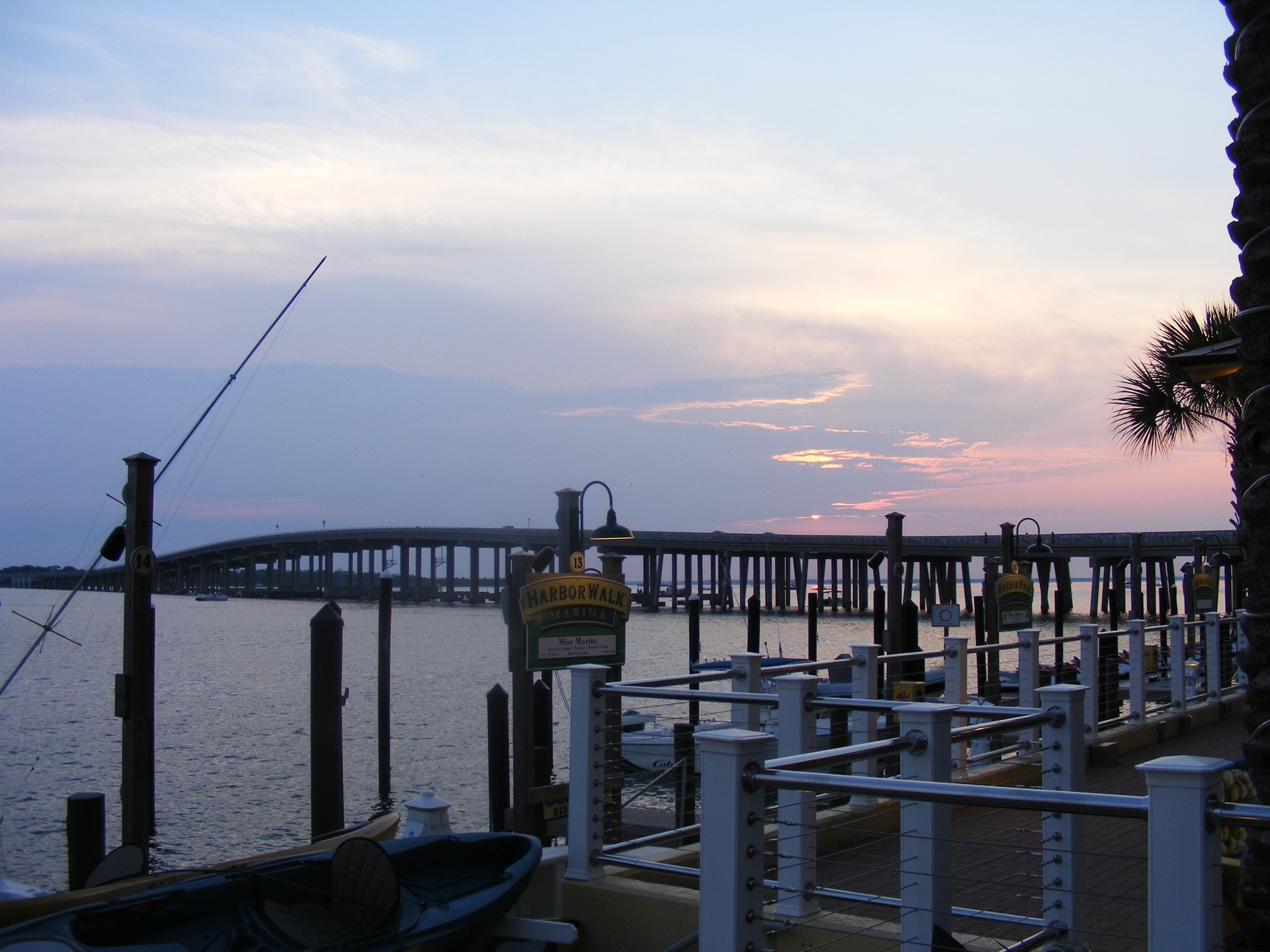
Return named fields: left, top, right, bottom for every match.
left=1012, top=515, right=1055, bottom=575
left=574, top=479, right=639, bottom=578
left=1197, top=535, right=1233, bottom=571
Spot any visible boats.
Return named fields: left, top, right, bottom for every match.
left=912, top=584, right=920, bottom=591
left=818, top=591, right=844, bottom=606
left=814, top=586, right=831, bottom=591
left=195, top=594, right=228, bottom=601
left=785, top=584, right=798, bottom=591
left=0, top=829, right=546, bottom=952
left=627, top=584, right=687, bottom=604
left=703, top=586, right=712, bottom=592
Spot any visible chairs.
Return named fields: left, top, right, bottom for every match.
left=325, top=837, right=401, bottom=920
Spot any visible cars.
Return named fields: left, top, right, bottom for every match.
left=762, top=532, right=777, bottom=535
left=711, top=530, right=726, bottom=533
left=502, top=526, right=514, bottom=529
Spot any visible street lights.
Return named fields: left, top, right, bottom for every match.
left=552, top=479, right=633, bottom=579
left=1191, top=534, right=1231, bottom=660
left=982, top=518, right=1053, bottom=770
